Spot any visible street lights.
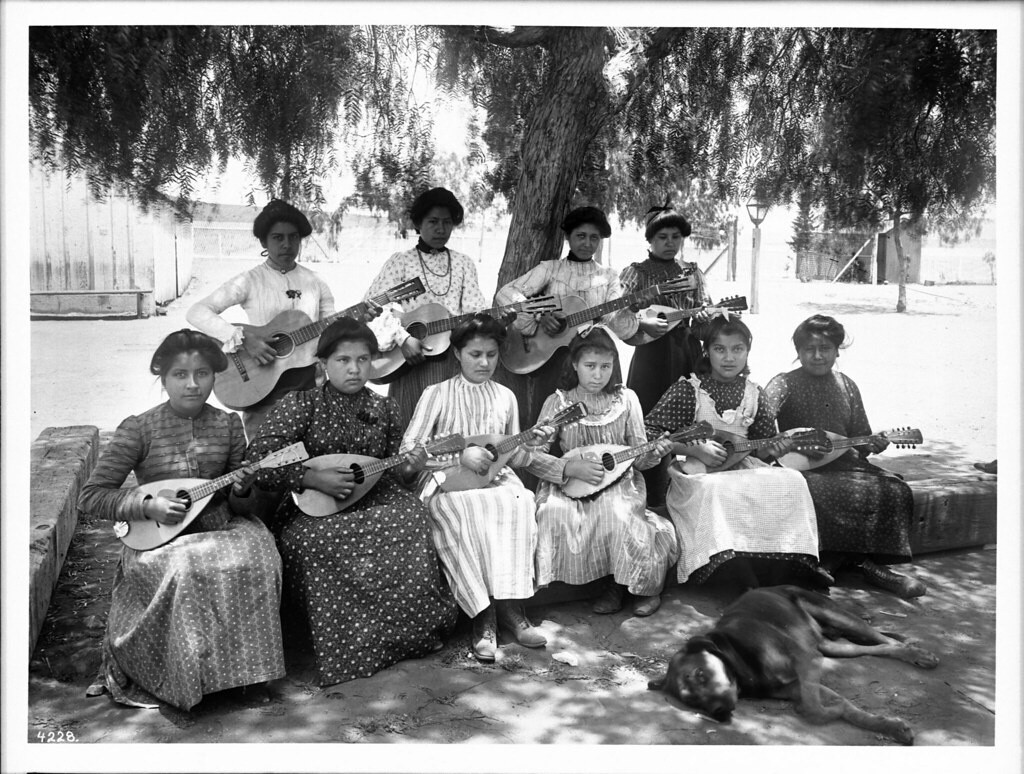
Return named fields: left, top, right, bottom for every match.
left=747, top=191, right=769, bottom=314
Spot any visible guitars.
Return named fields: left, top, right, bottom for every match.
left=772, top=426, right=924, bottom=473
left=621, top=295, right=749, bottom=347
left=114, top=440, right=310, bottom=552
left=555, top=419, right=715, bottom=501
left=676, top=429, right=829, bottom=475
left=211, top=275, right=427, bottom=411
left=495, top=273, right=698, bottom=379
left=367, top=291, right=564, bottom=386
left=432, top=400, right=590, bottom=493
left=290, top=430, right=467, bottom=519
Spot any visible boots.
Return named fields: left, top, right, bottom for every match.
left=472, top=597, right=499, bottom=660
left=500, top=596, right=545, bottom=646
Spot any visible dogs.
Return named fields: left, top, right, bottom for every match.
left=647, top=583, right=940, bottom=746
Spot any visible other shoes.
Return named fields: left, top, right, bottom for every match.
left=974, top=460, right=997, bottom=474
left=632, top=592, right=661, bottom=616
left=811, top=550, right=839, bottom=587
left=593, top=581, right=625, bottom=613
left=860, top=558, right=926, bottom=597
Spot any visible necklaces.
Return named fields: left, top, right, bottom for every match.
left=416, top=248, right=452, bottom=295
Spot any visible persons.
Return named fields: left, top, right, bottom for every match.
left=76, top=329, right=285, bottom=704
left=185, top=200, right=382, bottom=443
left=642, top=317, right=820, bottom=597
left=359, top=187, right=518, bottom=446
left=399, top=313, right=556, bottom=663
left=244, top=316, right=453, bottom=676
left=494, top=206, right=654, bottom=436
left=527, top=326, right=674, bottom=616
left=763, top=315, right=926, bottom=598
left=615, top=209, right=715, bottom=428
